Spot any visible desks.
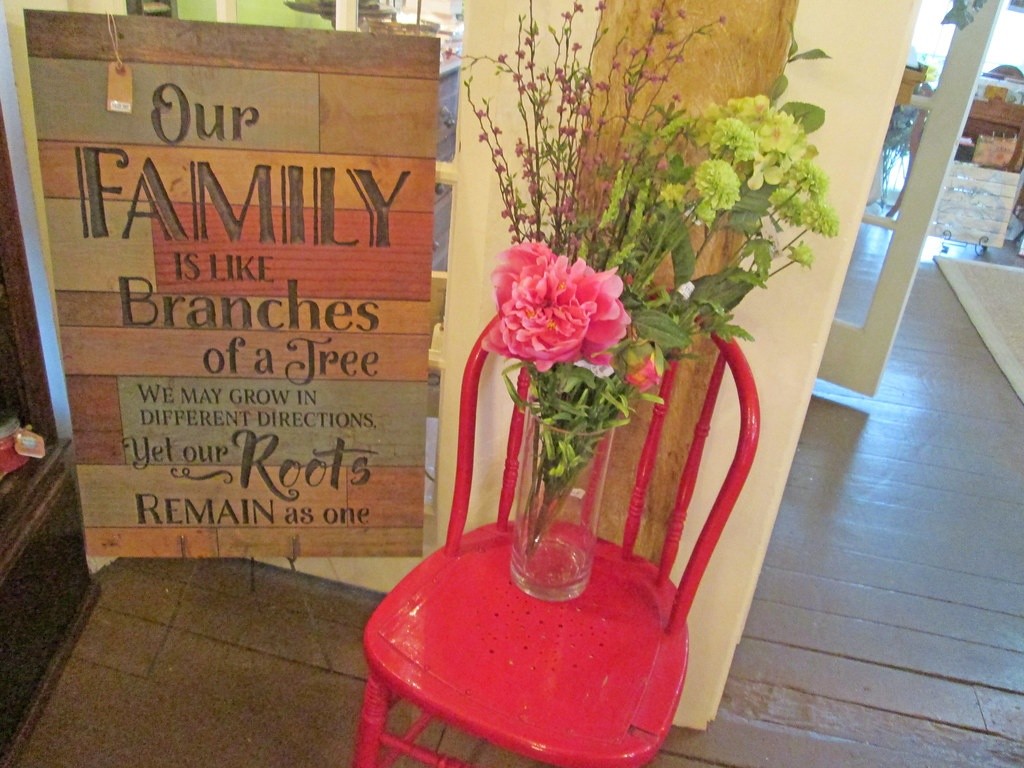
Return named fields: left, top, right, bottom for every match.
left=884, top=93, right=1024, bottom=218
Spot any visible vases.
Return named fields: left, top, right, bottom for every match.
left=512, top=402, right=615, bottom=602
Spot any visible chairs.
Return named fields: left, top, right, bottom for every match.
left=354, top=311, right=761, bottom=768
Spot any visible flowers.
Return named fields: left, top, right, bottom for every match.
left=444, top=0, right=838, bottom=561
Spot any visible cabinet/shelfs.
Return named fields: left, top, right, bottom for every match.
left=0, top=437, right=102, bottom=768
